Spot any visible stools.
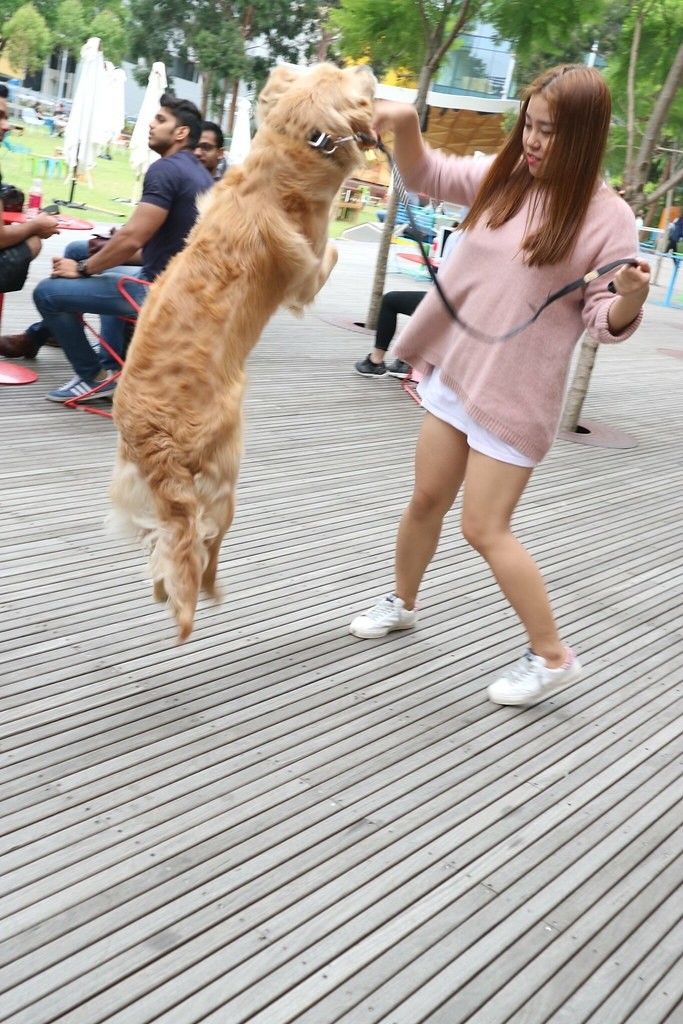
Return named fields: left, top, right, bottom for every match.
left=37, top=158, right=64, bottom=182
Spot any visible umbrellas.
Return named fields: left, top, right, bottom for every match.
left=61, top=33, right=171, bottom=178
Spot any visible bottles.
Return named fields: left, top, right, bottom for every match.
left=27, top=180, right=42, bottom=219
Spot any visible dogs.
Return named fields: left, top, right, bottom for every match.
left=109, top=60, right=422, bottom=645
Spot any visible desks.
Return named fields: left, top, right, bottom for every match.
left=0, top=208, right=93, bottom=385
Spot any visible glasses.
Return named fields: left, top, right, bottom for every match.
left=190, top=142, right=220, bottom=151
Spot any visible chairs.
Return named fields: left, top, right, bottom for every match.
left=62, top=278, right=151, bottom=421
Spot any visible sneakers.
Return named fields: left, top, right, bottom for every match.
left=386, top=358, right=413, bottom=378
left=45, top=369, right=120, bottom=402
left=353, top=352, right=387, bottom=378
left=348, top=592, right=418, bottom=639
left=486, top=645, right=582, bottom=705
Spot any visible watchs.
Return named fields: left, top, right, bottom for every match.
left=76, top=259, right=92, bottom=279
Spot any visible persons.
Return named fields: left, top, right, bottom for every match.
left=1, top=84, right=60, bottom=387
left=32, top=101, right=60, bottom=138
left=353, top=291, right=428, bottom=380
left=348, top=64, right=652, bottom=708
left=635, top=208, right=644, bottom=232
left=663, top=214, right=683, bottom=266
left=0, top=94, right=236, bottom=406
left=54, top=103, right=67, bottom=137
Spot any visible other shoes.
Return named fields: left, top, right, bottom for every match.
left=44, top=333, right=62, bottom=348
left=0, top=333, right=40, bottom=359
